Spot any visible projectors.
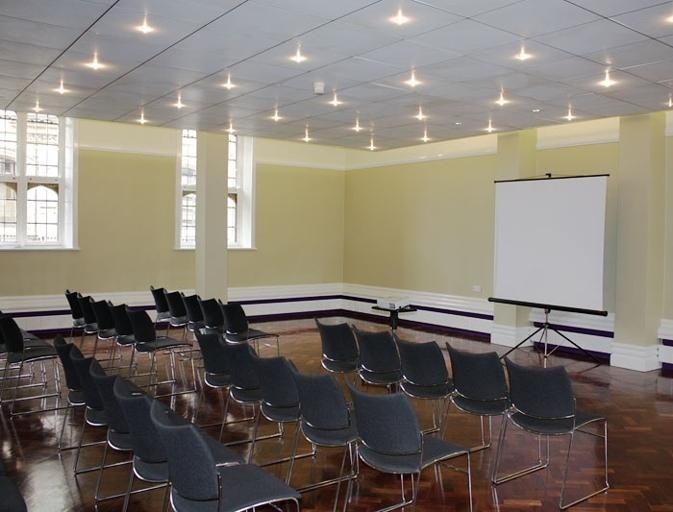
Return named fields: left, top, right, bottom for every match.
left=377, top=294, right=410, bottom=310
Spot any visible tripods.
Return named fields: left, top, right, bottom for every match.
left=499, top=310, right=602, bottom=369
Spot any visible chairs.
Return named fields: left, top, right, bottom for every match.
left=392, top=332, right=452, bottom=438
left=76, top=294, right=94, bottom=347
left=163, top=289, right=188, bottom=335
left=286, top=365, right=360, bottom=500
left=181, top=292, right=202, bottom=331
left=150, top=399, right=302, bottom=512
left=247, top=349, right=317, bottom=465
left=89, top=299, right=113, bottom=358
left=341, top=374, right=473, bottom=512
left=150, top=285, right=170, bottom=330
left=193, top=327, right=256, bottom=428
left=218, top=299, right=279, bottom=357
left=89, top=360, right=171, bottom=501
left=64, top=289, right=85, bottom=340
left=107, top=302, right=128, bottom=366
left=124, top=305, right=198, bottom=398
left=53, top=335, right=107, bottom=451
left=494, top=356, right=609, bottom=510
left=352, top=323, right=400, bottom=394
left=218, top=335, right=284, bottom=446
left=69, top=343, right=132, bottom=473
left=114, top=376, right=244, bottom=512
left=1, top=312, right=70, bottom=417
left=440, top=341, right=509, bottom=478
left=197, top=295, right=223, bottom=330
left=314, top=317, right=358, bottom=375
